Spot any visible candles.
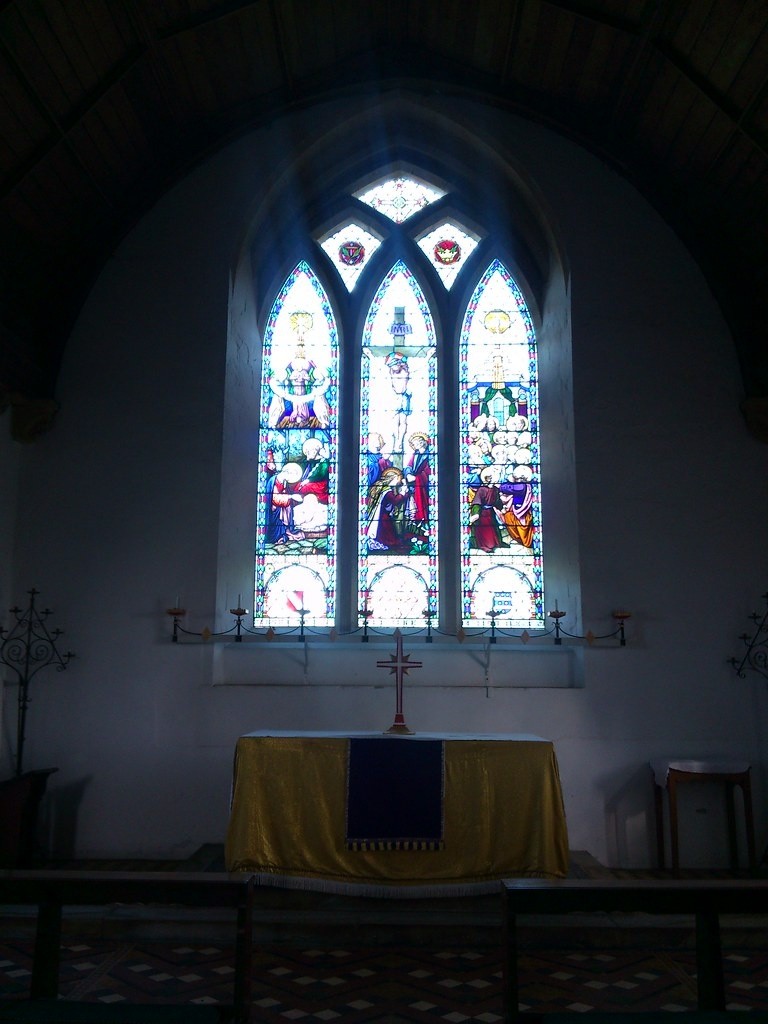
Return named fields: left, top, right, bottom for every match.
left=427, top=596, right=431, bottom=612
left=364, top=596, right=368, bottom=612
left=237, top=593, right=242, bottom=609
left=175, top=593, right=180, bottom=608
left=492, top=596, right=496, bottom=611
left=555, top=599, right=558, bottom=612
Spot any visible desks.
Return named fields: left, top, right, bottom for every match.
left=222, top=728, right=571, bottom=882
left=649, top=755, right=757, bottom=876
